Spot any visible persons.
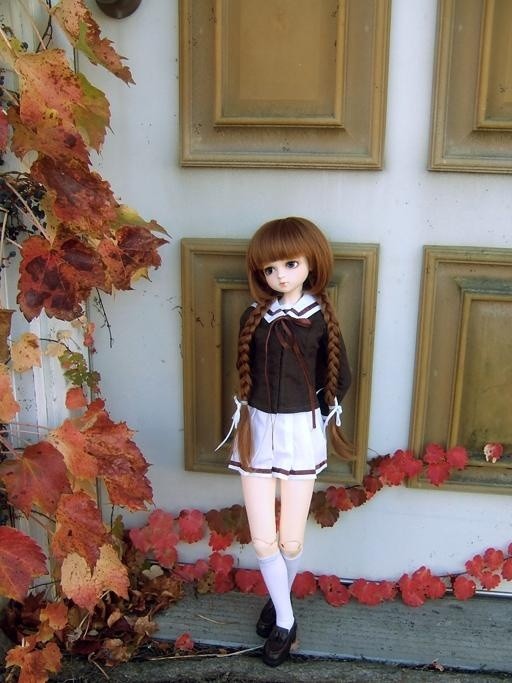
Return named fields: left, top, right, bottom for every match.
left=214, top=216, right=354, bottom=669
